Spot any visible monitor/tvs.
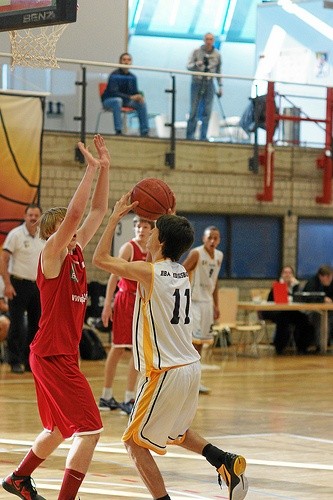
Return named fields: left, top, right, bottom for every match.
left=0, top=0, right=77, bottom=31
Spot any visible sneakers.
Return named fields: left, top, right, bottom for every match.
left=99, top=397, right=120, bottom=410
left=2, top=473, right=47, bottom=500
left=218, top=452, right=248, bottom=500
left=120, top=399, right=136, bottom=416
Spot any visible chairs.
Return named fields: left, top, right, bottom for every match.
left=95, top=83, right=138, bottom=137
left=211, top=287, right=271, bottom=357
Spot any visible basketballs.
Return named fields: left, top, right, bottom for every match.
left=130, top=177, right=174, bottom=220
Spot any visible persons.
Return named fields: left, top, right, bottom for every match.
left=0, top=276, right=10, bottom=343
left=98, top=214, right=156, bottom=415
left=101, top=52, right=150, bottom=136
left=304, top=267, right=333, bottom=351
left=93, top=196, right=250, bottom=500
left=186, top=32, right=223, bottom=142
left=1, top=204, right=47, bottom=372
left=179, top=226, right=224, bottom=392
left=262, top=266, right=320, bottom=353
left=2, top=134, right=111, bottom=500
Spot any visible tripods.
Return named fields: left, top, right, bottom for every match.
left=188, top=66, right=232, bottom=144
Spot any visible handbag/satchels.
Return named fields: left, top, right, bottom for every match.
left=273, top=279, right=288, bottom=303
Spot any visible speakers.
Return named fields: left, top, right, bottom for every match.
left=79, top=328, right=107, bottom=361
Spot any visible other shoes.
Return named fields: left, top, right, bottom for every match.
left=12, top=364, right=25, bottom=372
left=199, top=384, right=211, bottom=394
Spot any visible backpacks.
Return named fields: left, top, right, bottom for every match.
left=80, top=329, right=107, bottom=360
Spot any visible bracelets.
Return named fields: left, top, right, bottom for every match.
left=219, top=84, right=222, bottom=87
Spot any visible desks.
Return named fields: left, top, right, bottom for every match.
left=237, top=300, right=333, bottom=357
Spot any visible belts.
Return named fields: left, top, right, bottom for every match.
left=12, top=275, right=36, bottom=284
